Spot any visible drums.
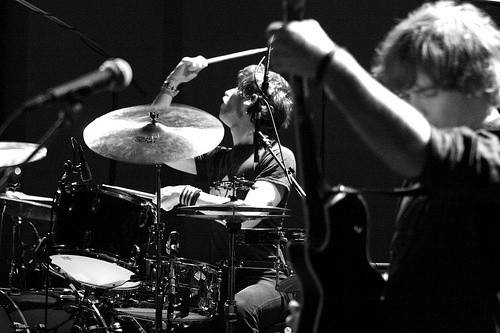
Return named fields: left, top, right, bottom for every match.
left=55, top=184, right=156, bottom=267
left=166, top=258, right=220, bottom=314
left=0, top=288, right=113, bottom=333
left=48, top=255, right=142, bottom=292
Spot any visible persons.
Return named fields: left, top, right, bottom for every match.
left=151, top=55, right=298, bottom=332
left=265, top=1, right=500, bottom=332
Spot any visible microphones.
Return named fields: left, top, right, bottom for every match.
left=166, top=231, right=180, bottom=255
left=23, top=58, right=133, bottom=113
left=73, top=137, right=92, bottom=182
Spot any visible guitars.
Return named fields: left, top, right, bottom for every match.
left=280, top=0, right=387, bottom=333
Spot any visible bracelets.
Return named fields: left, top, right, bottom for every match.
left=315, top=46, right=352, bottom=87
left=160, top=80, right=180, bottom=97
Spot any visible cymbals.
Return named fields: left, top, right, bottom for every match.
left=0, top=141, right=47, bottom=167
left=275, top=262, right=390, bottom=292
left=83, top=105, right=225, bottom=163
left=178, top=213, right=292, bottom=220
left=178, top=203, right=292, bottom=213
